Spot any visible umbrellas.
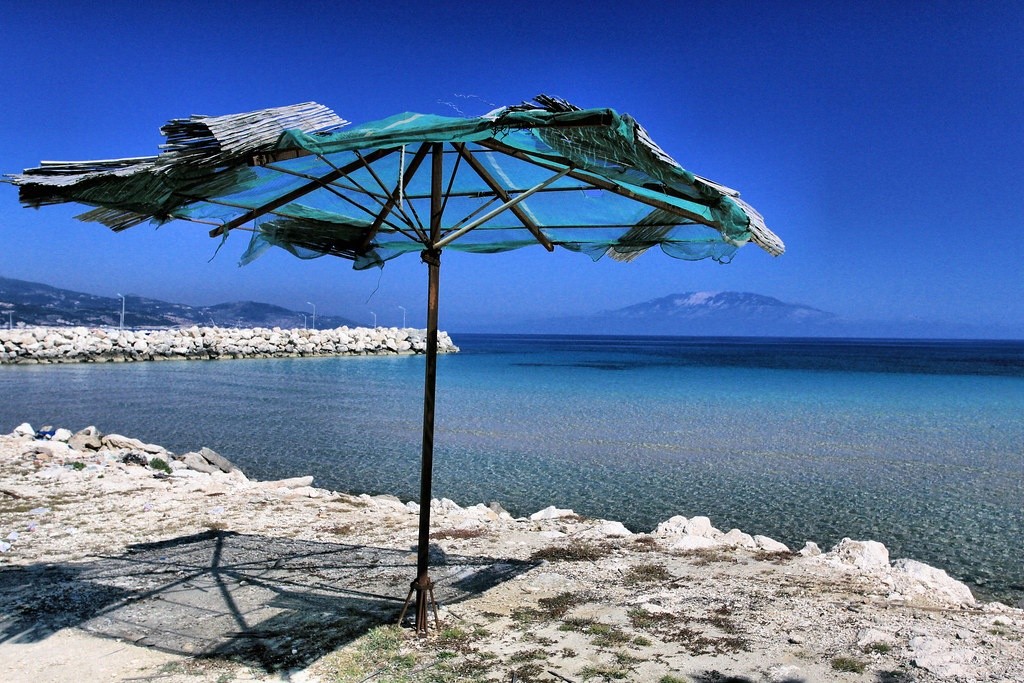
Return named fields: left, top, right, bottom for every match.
left=21, top=108, right=785, bottom=633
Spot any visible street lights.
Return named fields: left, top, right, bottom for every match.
left=118, top=312, right=122, bottom=329
left=307, top=302, right=315, bottom=329
left=370, top=312, right=377, bottom=329
left=116, top=293, right=124, bottom=330
left=398, top=306, right=405, bottom=328
left=302, top=314, right=306, bottom=328
